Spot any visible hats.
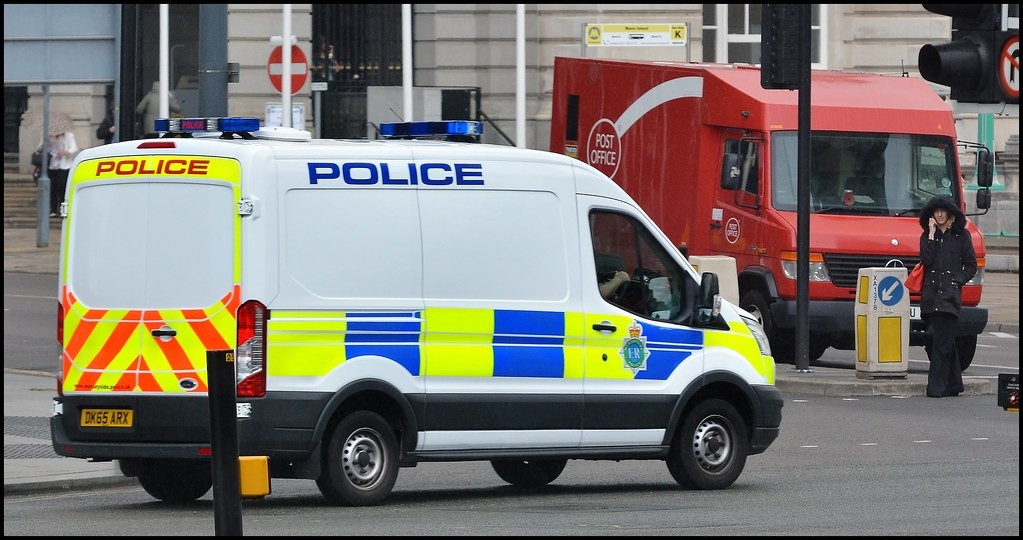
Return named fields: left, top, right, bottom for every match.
left=109, top=99, right=114, bottom=110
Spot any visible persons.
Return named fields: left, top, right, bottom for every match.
left=96, top=100, right=115, bottom=144
left=35, top=113, right=79, bottom=217
left=136, top=82, right=182, bottom=137
left=599, top=269, right=630, bottom=300
left=905, top=199, right=977, bottom=397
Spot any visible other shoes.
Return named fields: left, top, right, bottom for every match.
left=946, top=382, right=964, bottom=396
left=49, top=213, right=58, bottom=218
left=927, top=387, right=946, bottom=397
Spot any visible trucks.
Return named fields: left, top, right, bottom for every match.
left=551, top=55, right=994, bottom=365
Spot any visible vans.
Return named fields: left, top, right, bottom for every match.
left=50, top=113, right=787, bottom=509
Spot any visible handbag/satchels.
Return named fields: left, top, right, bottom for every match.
left=904, top=261, right=925, bottom=292
left=31, top=151, right=51, bottom=165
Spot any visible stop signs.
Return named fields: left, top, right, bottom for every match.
left=267, top=42, right=309, bottom=94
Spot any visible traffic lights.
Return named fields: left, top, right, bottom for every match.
left=919, top=2, right=1002, bottom=106
